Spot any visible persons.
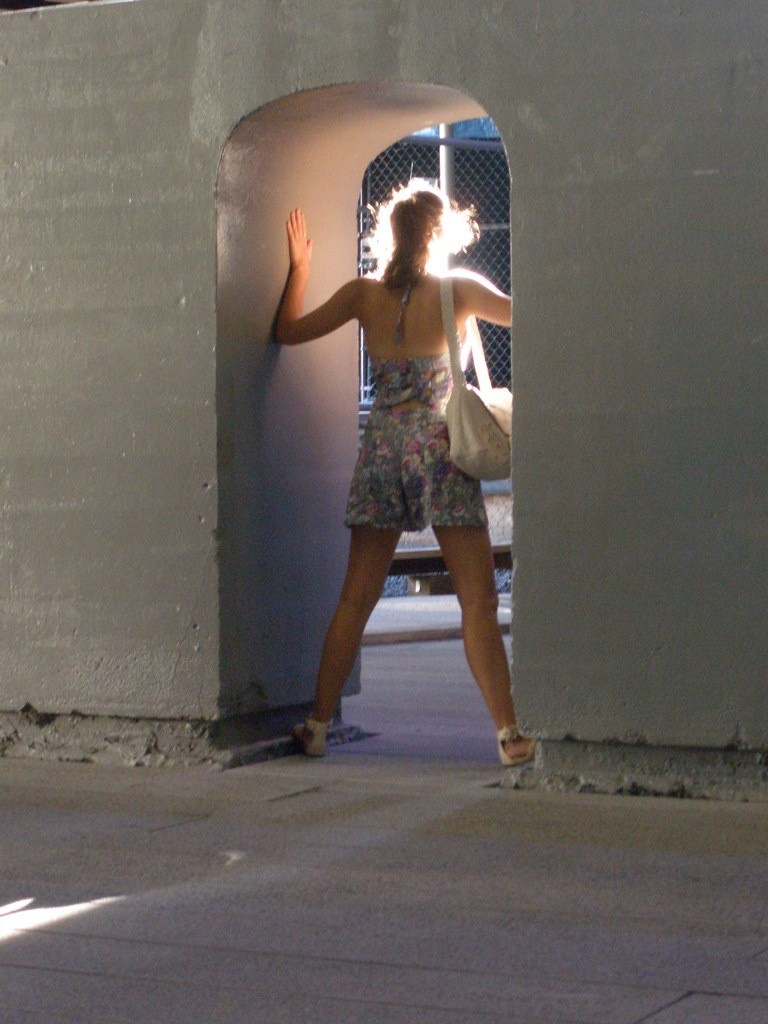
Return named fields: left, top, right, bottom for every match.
left=273, top=188, right=536, bottom=768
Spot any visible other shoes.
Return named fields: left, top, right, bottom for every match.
left=291, top=712, right=333, bottom=756
left=496, top=724, right=541, bottom=765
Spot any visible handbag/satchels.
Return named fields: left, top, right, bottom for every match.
left=439, top=275, right=511, bottom=480
